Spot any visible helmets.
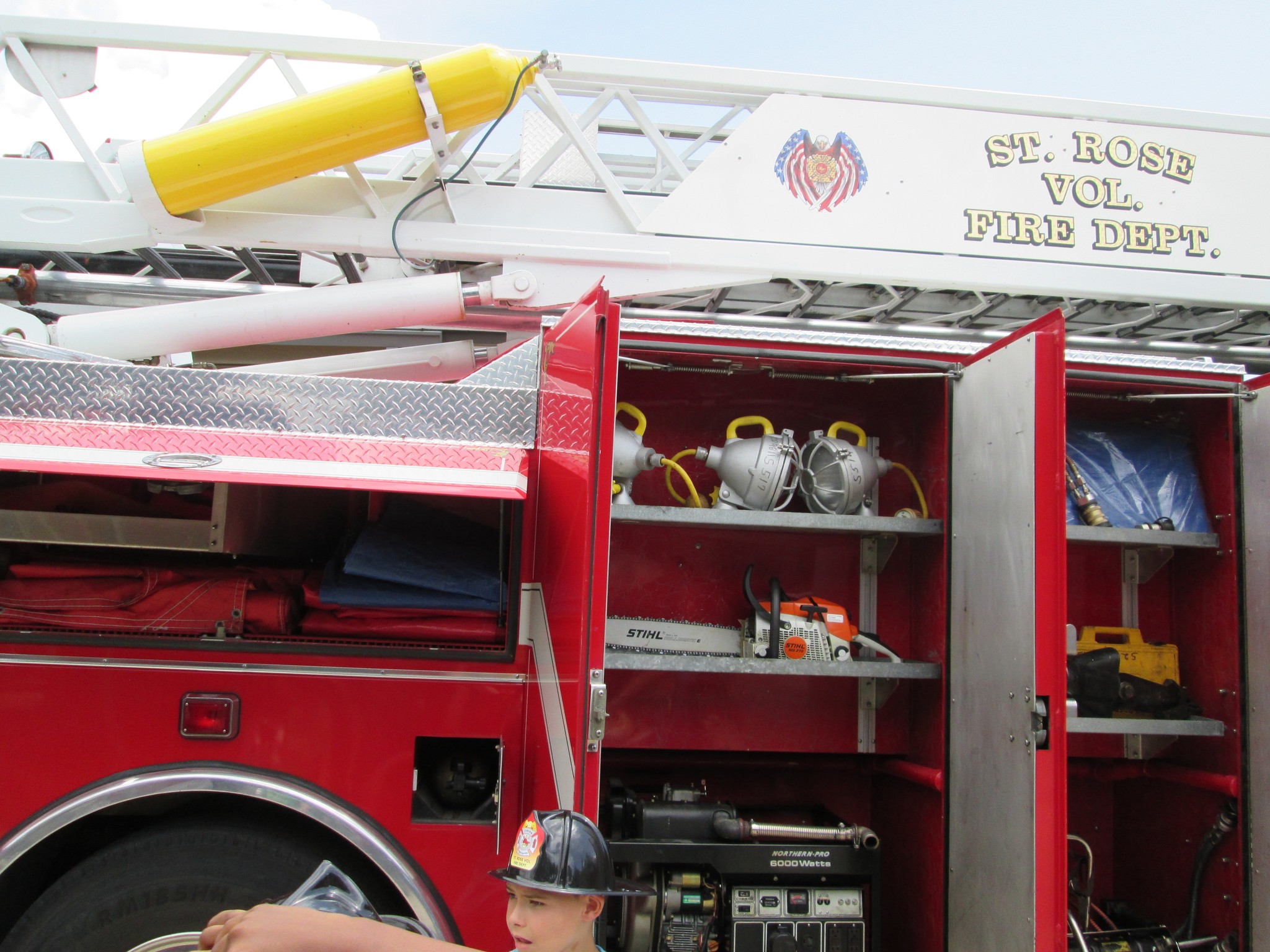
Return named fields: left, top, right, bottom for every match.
left=486, top=808, right=662, bottom=897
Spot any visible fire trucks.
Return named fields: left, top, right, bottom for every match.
left=0, top=13, right=1270, bottom=952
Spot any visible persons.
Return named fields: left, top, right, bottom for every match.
left=489, top=805, right=659, bottom=952
left=197, top=901, right=485, bottom=952
